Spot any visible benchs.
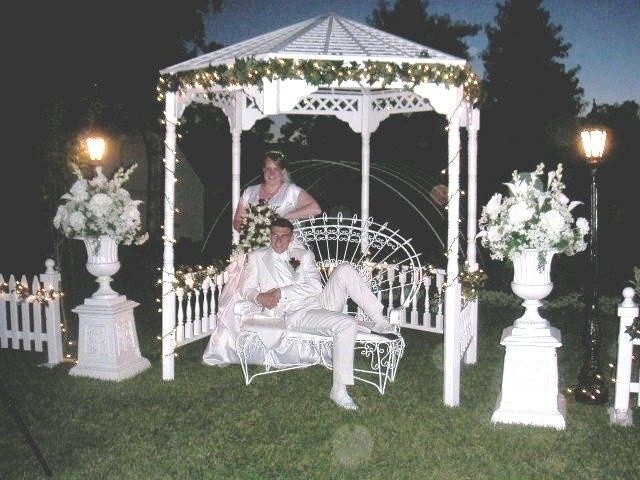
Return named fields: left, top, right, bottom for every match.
left=235, top=216, right=424, bottom=397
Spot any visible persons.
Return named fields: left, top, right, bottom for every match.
left=203, top=144, right=322, bottom=366
left=238, top=218, right=399, bottom=410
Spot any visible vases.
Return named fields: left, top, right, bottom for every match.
left=74, top=232, right=122, bottom=301
left=504, top=247, right=557, bottom=327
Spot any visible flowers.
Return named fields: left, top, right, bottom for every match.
left=481, top=162, right=588, bottom=266
left=51, top=165, right=149, bottom=248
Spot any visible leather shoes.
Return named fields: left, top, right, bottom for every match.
left=372, top=323, right=398, bottom=340
left=329, top=388, right=359, bottom=409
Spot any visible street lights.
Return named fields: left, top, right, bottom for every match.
left=84, top=132, right=110, bottom=180
left=565, top=98, right=615, bottom=405
